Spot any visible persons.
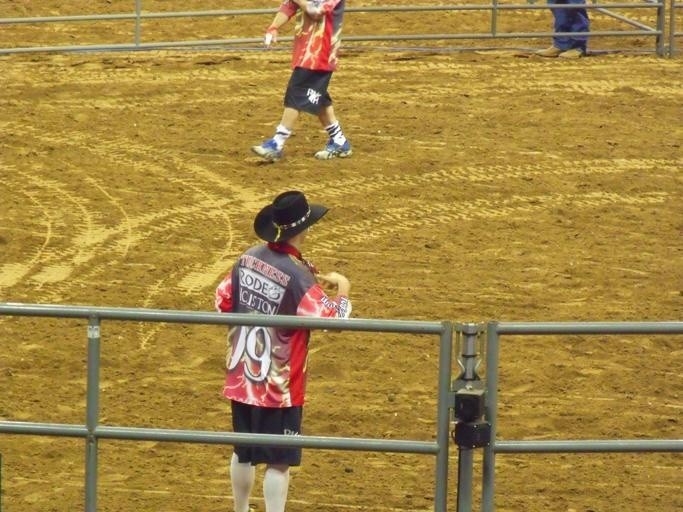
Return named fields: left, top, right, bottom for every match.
left=535, top=0, right=590, bottom=59
left=215, top=190, right=352, bottom=512
left=248, top=0, right=352, bottom=162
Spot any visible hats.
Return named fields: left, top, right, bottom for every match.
left=253, top=189, right=328, bottom=241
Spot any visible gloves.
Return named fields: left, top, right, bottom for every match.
left=264, top=24, right=278, bottom=48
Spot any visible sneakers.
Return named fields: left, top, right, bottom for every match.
left=314, top=139, right=353, bottom=160
left=535, top=45, right=565, bottom=56
left=559, top=48, right=583, bottom=58
left=251, top=139, right=281, bottom=162
